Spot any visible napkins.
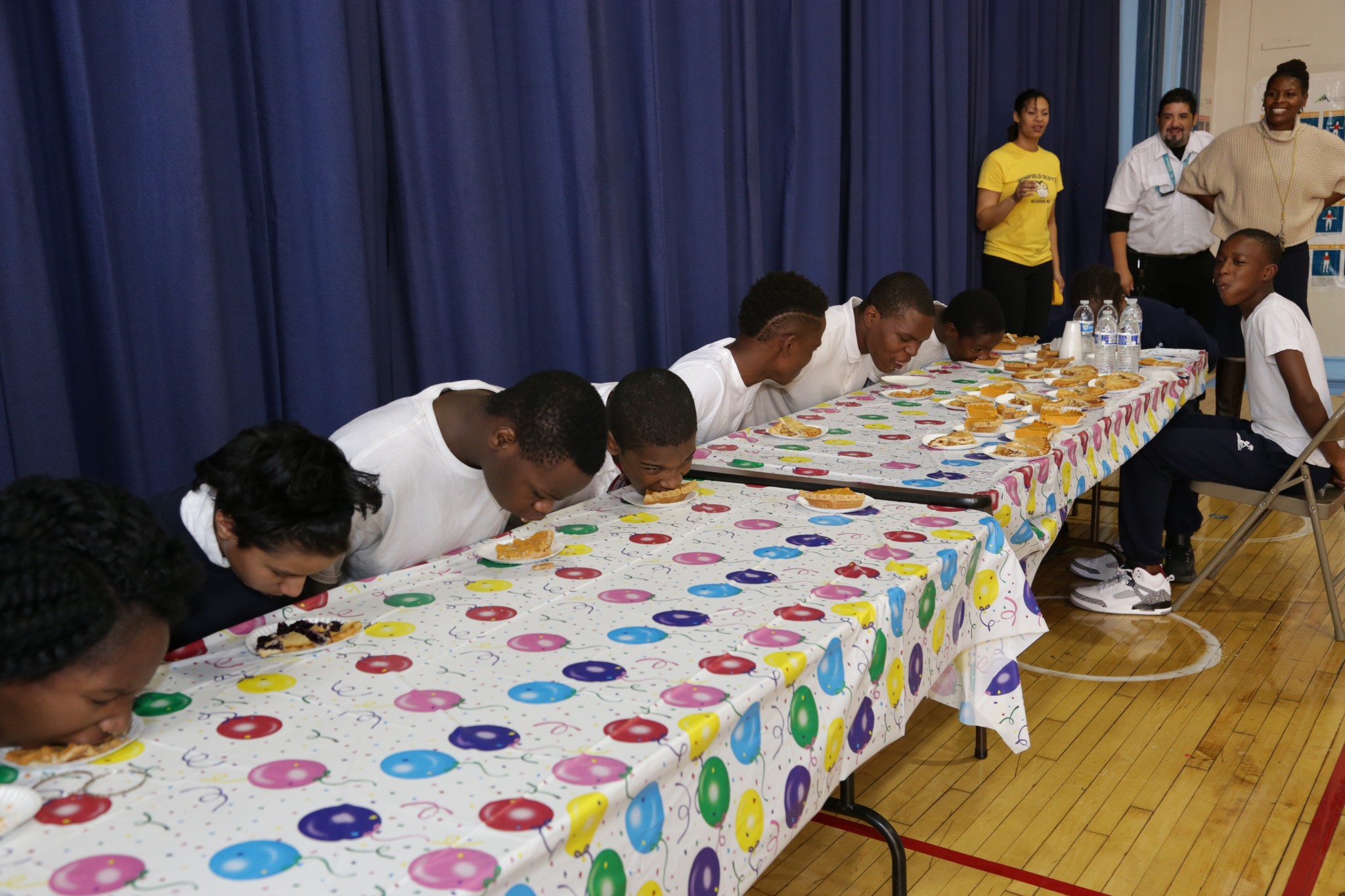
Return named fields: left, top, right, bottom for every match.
left=1138, top=367, right=1180, bottom=381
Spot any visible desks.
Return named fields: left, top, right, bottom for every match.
left=688, top=349, right=1208, bottom=586
left=0, top=487, right=1046, bottom=896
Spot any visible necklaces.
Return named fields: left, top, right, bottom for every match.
left=1261, top=131, right=1296, bottom=254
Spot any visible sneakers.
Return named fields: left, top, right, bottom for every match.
left=1073, top=569, right=1175, bottom=615
left=1162, top=531, right=1198, bottom=583
left=1072, top=540, right=1125, bottom=582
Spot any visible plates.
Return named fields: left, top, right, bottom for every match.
left=984, top=444, right=1054, bottom=461
left=881, top=376, right=932, bottom=386
left=623, top=490, right=698, bottom=508
left=1044, top=368, right=1101, bottom=389
left=952, top=423, right=1073, bottom=444
left=1088, top=373, right=1147, bottom=393
left=0, top=784, right=44, bottom=836
left=880, top=389, right=934, bottom=399
left=0, top=711, right=144, bottom=769
left=796, top=492, right=874, bottom=513
left=477, top=538, right=564, bottom=564
left=246, top=616, right=362, bottom=657
left=967, top=382, right=1106, bottom=416
left=1138, top=358, right=1186, bottom=369
left=992, top=349, right=1024, bottom=354
left=765, top=425, right=829, bottom=440
left=1031, top=342, right=1052, bottom=351
left=938, top=398, right=995, bottom=410
left=922, top=433, right=987, bottom=449
left=963, top=412, right=1085, bottom=428
left=997, top=353, right=1077, bottom=382
left=957, top=361, right=1004, bottom=369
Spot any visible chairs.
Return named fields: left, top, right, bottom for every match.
left=1169, top=401, right=1345, bottom=644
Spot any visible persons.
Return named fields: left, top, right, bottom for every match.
left=667, top=267, right=1009, bottom=449
left=1, top=474, right=184, bottom=747
left=137, top=420, right=386, bottom=656
left=1068, top=229, right=1345, bottom=619
left=304, top=367, right=610, bottom=600
left=551, top=370, right=700, bottom=514
left=1107, top=61, right=1345, bottom=420
left=974, top=86, right=1068, bottom=342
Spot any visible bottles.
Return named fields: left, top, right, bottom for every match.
left=1097, top=300, right=1118, bottom=327
left=1073, top=300, right=1093, bottom=364
left=1093, top=310, right=1116, bottom=373
left=1115, top=298, right=1142, bottom=374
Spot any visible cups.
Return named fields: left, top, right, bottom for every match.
left=1059, top=321, right=1082, bottom=364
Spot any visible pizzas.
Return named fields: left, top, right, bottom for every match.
left=945, top=333, right=1144, bottom=441
left=644, top=479, right=698, bottom=504
left=1138, top=357, right=1185, bottom=366
left=5, top=736, right=131, bottom=766
left=769, top=417, right=821, bottom=437
left=495, top=529, right=554, bottom=570
left=888, top=387, right=935, bottom=398
left=256, top=619, right=363, bottom=659
left=927, top=430, right=977, bottom=448
left=994, top=436, right=1050, bottom=457
left=798, top=487, right=866, bottom=509
